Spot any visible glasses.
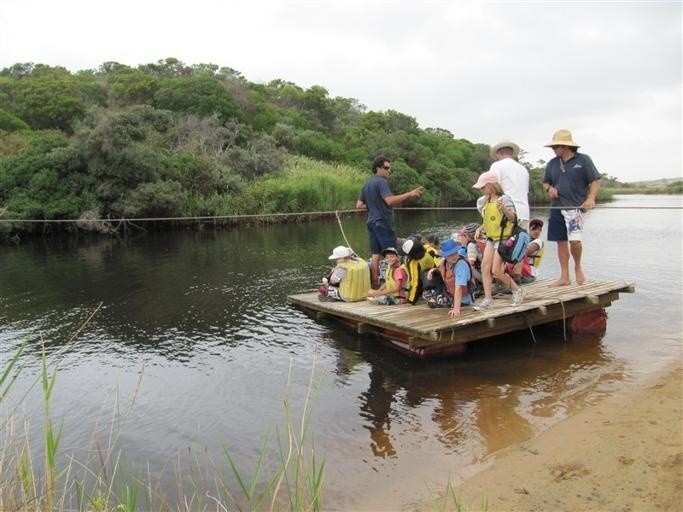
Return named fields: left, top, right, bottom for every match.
left=552, top=145, right=560, bottom=149
left=382, top=165, right=391, bottom=170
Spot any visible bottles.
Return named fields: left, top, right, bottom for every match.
left=506, top=235, right=516, bottom=247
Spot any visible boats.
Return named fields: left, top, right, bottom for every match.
left=286, top=268, right=634, bottom=357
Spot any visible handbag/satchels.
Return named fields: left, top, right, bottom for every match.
left=475, top=229, right=489, bottom=244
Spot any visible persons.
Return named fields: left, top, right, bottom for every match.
left=369, top=217, right=544, bottom=318
left=541, top=128, right=602, bottom=287
left=470, top=172, right=523, bottom=311
left=489, top=140, right=529, bottom=236
left=317, top=245, right=368, bottom=303
left=357, top=156, right=425, bottom=289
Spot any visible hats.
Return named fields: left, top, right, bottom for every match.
left=544, top=129, right=581, bottom=148
left=382, top=247, right=398, bottom=256
left=436, top=238, right=465, bottom=256
left=327, top=246, right=351, bottom=259
left=530, top=219, right=544, bottom=227
left=458, top=223, right=480, bottom=236
left=472, top=172, right=499, bottom=189
left=489, top=142, right=520, bottom=159
left=396, top=235, right=421, bottom=255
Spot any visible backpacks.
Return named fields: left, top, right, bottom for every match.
left=497, top=213, right=531, bottom=264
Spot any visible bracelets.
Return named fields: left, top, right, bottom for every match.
left=545, top=185, right=551, bottom=194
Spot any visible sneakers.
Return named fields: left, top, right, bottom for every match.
left=472, top=284, right=527, bottom=311
left=319, top=295, right=337, bottom=302
